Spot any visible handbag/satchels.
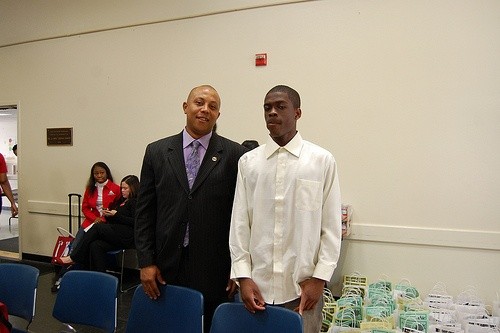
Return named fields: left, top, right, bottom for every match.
left=341, top=204, right=353, bottom=236
left=320, top=270, right=500, bottom=333
left=51, top=227, right=76, bottom=264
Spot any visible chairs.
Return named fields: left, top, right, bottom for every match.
left=211, top=303, right=303, bottom=333
left=51, top=270, right=119, bottom=333
left=124, top=283, right=204, bottom=333
left=0, top=262, right=40, bottom=333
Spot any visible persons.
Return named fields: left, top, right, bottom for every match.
left=55, top=175, right=139, bottom=271
left=12, top=144, right=17, bottom=156
left=229, top=85, right=343, bottom=333
left=134, top=85, right=251, bottom=333
left=0, top=153, right=18, bottom=218
left=51, top=162, right=121, bottom=293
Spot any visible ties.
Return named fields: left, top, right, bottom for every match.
left=181, top=140, right=201, bottom=247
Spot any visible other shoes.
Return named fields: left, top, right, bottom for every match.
left=51, top=281, right=61, bottom=292
left=53, top=256, right=75, bottom=265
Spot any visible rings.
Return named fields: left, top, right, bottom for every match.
left=97, top=221, right=98, bottom=222
